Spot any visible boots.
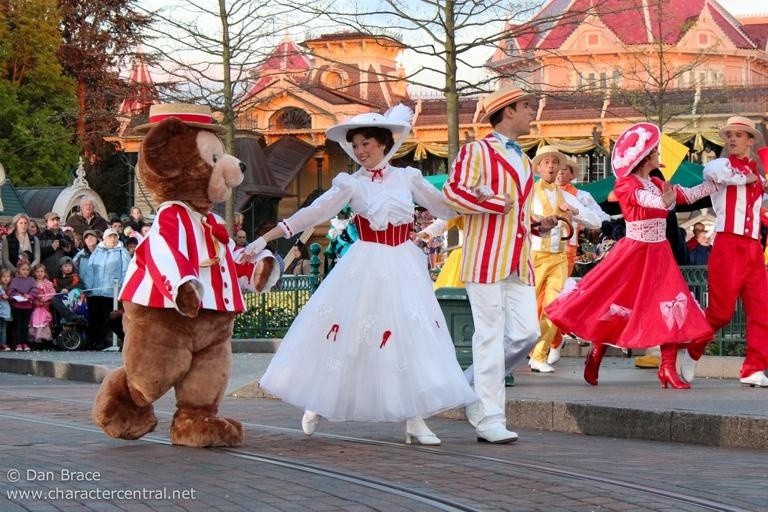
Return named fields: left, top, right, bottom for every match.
left=660, top=345, right=690, bottom=389
left=584, top=342, right=605, bottom=385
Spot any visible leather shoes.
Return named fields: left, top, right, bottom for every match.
left=530, top=360, right=554, bottom=374
left=476, top=422, right=518, bottom=444
left=549, top=339, right=566, bottom=363
left=740, top=370, right=768, bottom=388
left=681, top=348, right=697, bottom=384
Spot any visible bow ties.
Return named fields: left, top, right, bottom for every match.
left=541, top=182, right=556, bottom=190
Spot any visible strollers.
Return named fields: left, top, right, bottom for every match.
left=49, top=293, right=89, bottom=350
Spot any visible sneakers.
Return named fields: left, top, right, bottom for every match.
left=0, top=345, right=11, bottom=351
left=23, top=344, right=31, bottom=351
left=15, top=344, right=23, bottom=351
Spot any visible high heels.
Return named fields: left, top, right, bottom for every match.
left=301, top=409, right=320, bottom=435
left=402, top=417, right=442, bottom=447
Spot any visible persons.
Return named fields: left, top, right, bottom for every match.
left=73, top=230, right=106, bottom=351
left=292, top=240, right=312, bottom=274
left=68, top=196, right=108, bottom=235
left=234, top=229, right=248, bottom=251
left=126, top=237, right=139, bottom=256
left=443, top=84, right=542, bottom=443
left=1, top=268, right=12, bottom=350
left=681, top=117, right=763, bottom=385
left=124, top=207, right=147, bottom=231
left=547, top=155, right=612, bottom=365
left=232, top=212, right=244, bottom=235
left=62, top=226, right=73, bottom=238
left=3, top=214, right=41, bottom=274
left=544, top=123, right=715, bottom=390
left=52, top=256, right=88, bottom=324
left=88, top=232, right=131, bottom=351
left=27, top=220, right=40, bottom=235
left=142, top=224, right=150, bottom=235
left=73, top=232, right=81, bottom=251
left=1, top=236, right=4, bottom=267
left=4, top=223, right=14, bottom=234
left=601, top=167, right=713, bottom=369
left=35, top=212, right=78, bottom=280
left=686, top=222, right=704, bottom=249
left=109, top=218, right=128, bottom=245
left=689, top=232, right=712, bottom=266
left=27, top=264, right=56, bottom=350
left=86, top=228, right=87, bottom=230
left=7, top=260, right=36, bottom=350
left=411, top=215, right=465, bottom=290
left=527, top=146, right=603, bottom=374
left=241, top=112, right=477, bottom=445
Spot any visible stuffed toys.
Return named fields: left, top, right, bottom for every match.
left=94, top=103, right=247, bottom=449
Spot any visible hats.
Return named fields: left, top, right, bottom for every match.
left=719, top=116, right=764, bottom=139
left=479, top=85, right=533, bottom=122
left=132, top=103, right=230, bottom=132
left=103, top=229, right=118, bottom=238
left=533, top=145, right=567, bottom=171
left=45, top=212, right=60, bottom=222
left=566, top=161, right=581, bottom=176
left=59, top=256, right=74, bottom=267
left=326, top=105, right=413, bottom=172
left=613, top=123, right=660, bottom=180
left=83, top=230, right=97, bottom=237
